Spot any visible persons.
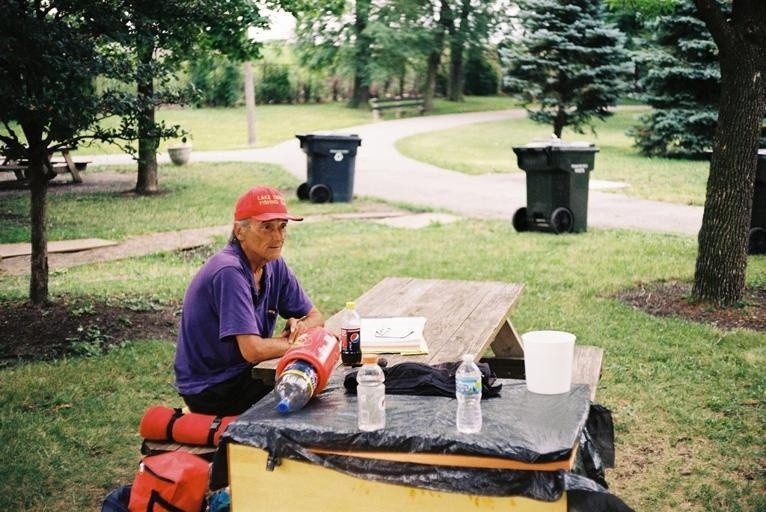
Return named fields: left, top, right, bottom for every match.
left=174, top=184, right=325, bottom=418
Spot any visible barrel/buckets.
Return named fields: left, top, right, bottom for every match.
left=521, top=329, right=575, bottom=394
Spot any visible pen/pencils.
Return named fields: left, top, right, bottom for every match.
left=284, top=315, right=309, bottom=332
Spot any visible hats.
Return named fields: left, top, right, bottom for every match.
left=235, top=186, right=304, bottom=222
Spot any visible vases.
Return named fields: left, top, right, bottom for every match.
left=169, top=147, right=191, bottom=165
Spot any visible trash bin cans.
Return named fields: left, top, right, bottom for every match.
left=749, top=150, right=766, bottom=255
left=513, top=144, right=600, bottom=235
left=295, top=135, right=362, bottom=202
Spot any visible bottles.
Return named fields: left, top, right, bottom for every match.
left=356, top=354, right=386, bottom=432
left=339, top=301, right=363, bottom=365
left=274, top=360, right=318, bottom=416
left=454, top=354, right=483, bottom=435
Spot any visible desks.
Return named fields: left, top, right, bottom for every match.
left=254, top=278, right=523, bottom=381
left=220, top=379, right=591, bottom=512
left=7, top=146, right=82, bottom=182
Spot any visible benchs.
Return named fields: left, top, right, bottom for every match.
left=572, top=345, right=603, bottom=403
left=370, top=96, right=425, bottom=119
left=145, top=405, right=222, bottom=455
left=19, top=160, right=93, bottom=164
left=0, top=166, right=29, bottom=170
